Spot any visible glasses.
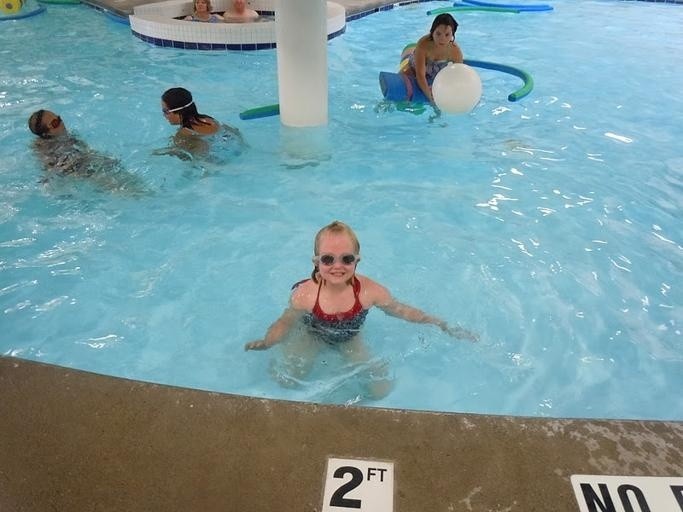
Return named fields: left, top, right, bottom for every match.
left=310, top=253, right=361, bottom=267
left=35, top=112, right=63, bottom=136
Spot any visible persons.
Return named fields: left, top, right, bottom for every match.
left=181, top=0, right=225, bottom=24
left=243, top=219, right=482, bottom=398
left=221, top=0, right=259, bottom=23
left=25, top=110, right=148, bottom=198
left=149, top=84, right=246, bottom=184
left=399, top=13, right=465, bottom=123
